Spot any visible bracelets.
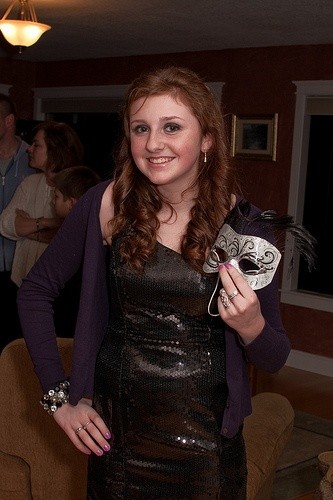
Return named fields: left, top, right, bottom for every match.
left=36, top=217, right=42, bottom=230
left=40, top=381, right=71, bottom=416
left=37, top=230, right=40, bottom=241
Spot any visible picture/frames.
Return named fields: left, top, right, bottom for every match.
left=228, top=112, right=279, bottom=162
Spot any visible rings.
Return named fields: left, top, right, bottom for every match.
left=83, top=421, right=91, bottom=430
left=75, top=427, right=83, bottom=435
left=228, top=290, right=239, bottom=299
left=220, top=290, right=231, bottom=309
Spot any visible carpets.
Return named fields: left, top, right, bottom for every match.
left=254, top=412, right=333, bottom=500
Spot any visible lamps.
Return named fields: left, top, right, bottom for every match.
left=0, top=0, right=51, bottom=55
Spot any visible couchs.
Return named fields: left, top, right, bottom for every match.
left=0, top=337, right=296, bottom=500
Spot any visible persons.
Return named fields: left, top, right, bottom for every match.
left=17, top=67, right=291, bottom=500
left=0, top=122, right=78, bottom=340
left=51, top=166, right=102, bottom=338
left=0, top=93, right=43, bottom=354
